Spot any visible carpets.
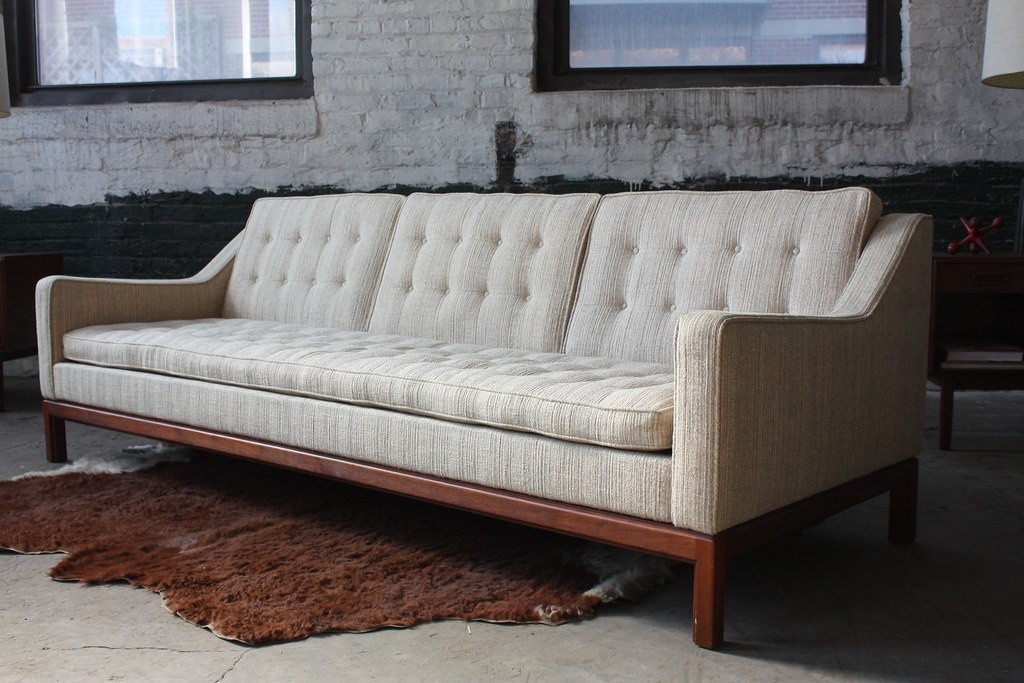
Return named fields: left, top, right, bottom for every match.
left=0, top=441, right=692, bottom=647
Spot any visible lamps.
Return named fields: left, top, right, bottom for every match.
left=981, top=0, right=1024, bottom=251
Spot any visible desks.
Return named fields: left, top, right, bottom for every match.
left=928, top=251, right=1024, bottom=451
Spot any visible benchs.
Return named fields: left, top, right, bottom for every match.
left=33, top=191, right=938, bottom=649
